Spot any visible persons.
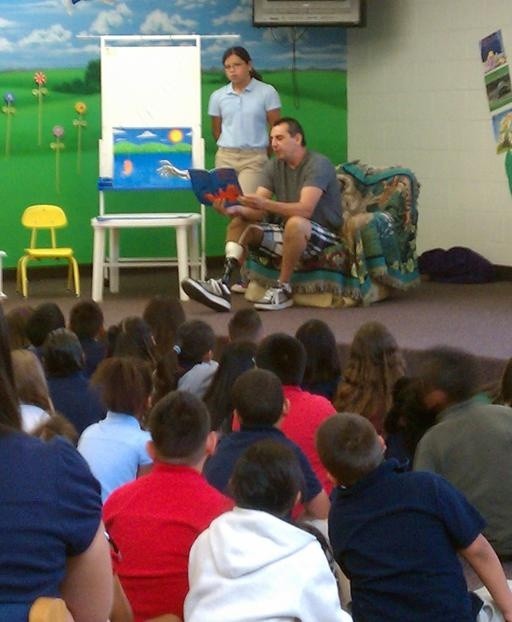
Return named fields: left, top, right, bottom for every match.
left=181, top=117, right=346, bottom=312
left=208, top=46, right=283, bottom=294
left=1, top=292, right=512, bottom=622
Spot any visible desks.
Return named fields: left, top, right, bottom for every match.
left=90, top=213, right=201, bottom=303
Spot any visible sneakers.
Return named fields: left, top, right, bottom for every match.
left=181, top=275, right=230, bottom=312
left=254, top=286, right=293, bottom=310
left=232, top=281, right=248, bottom=294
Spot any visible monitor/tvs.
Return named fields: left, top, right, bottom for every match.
left=252, top=0, right=367, bottom=28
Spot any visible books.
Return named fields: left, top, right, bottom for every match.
left=188, top=165, right=244, bottom=207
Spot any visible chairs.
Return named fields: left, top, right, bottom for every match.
left=16, top=204, right=80, bottom=298
left=243, top=158, right=422, bottom=308
